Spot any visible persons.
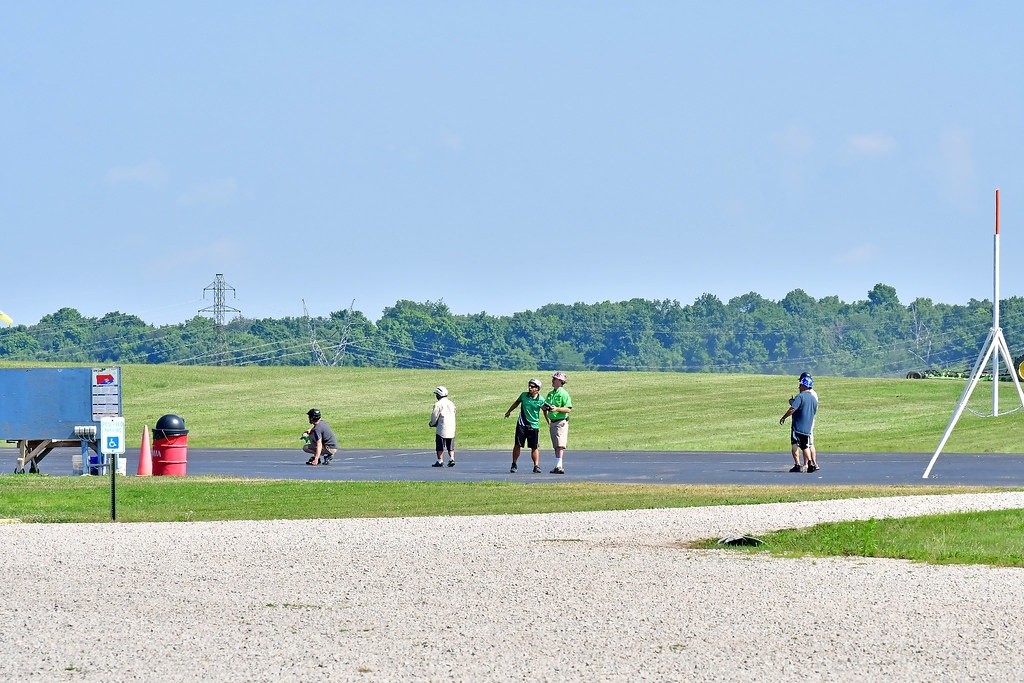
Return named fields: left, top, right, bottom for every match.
left=798, top=372, right=819, bottom=472
left=504, top=379, right=551, bottom=473
left=780, top=376, right=817, bottom=472
left=429, top=387, right=456, bottom=467
left=541, top=372, right=572, bottom=474
left=302, top=408, right=338, bottom=465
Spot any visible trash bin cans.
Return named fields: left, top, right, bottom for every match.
left=150, top=413, right=189, bottom=478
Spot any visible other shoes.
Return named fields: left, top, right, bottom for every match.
left=306, top=458, right=321, bottom=464
left=322, top=453, right=333, bottom=465
left=807, top=465, right=816, bottom=473
left=790, top=465, right=801, bottom=472
left=533, top=465, right=541, bottom=473
left=432, top=461, right=444, bottom=467
left=550, top=467, right=565, bottom=473
left=801, top=465, right=820, bottom=471
left=447, top=460, right=455, bottom=467
left=510, top=463, right=517, bottom=472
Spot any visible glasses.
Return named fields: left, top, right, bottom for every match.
left=529, top=385, right=537, bottom=389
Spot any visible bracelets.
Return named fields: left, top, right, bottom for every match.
left=304, top=431, right=308, bottom=434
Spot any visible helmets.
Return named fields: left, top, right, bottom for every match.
left=434, top=387, right=448, bottom=396
left=552, top=372, right=567, bottom=383
left=799, top=377, right=813, bottom=388
left=798, top=372, right=812, bottom=380
left=307, top=409, right=321, bottom=419
left=529, top=379, right=542, bottom=391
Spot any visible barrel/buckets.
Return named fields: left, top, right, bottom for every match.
left=152, top=428, right=189, bottom=476
